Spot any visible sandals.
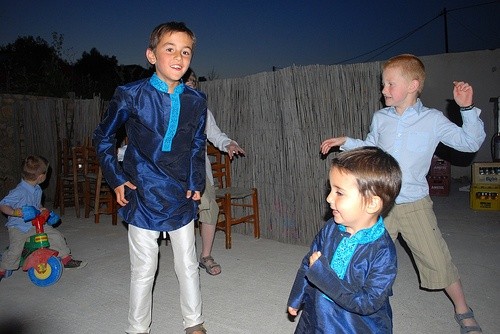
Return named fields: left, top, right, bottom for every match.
left=199, top=255, right=221, bottom=276
left=185, top=324, right=207, bottom=334
left=454, top=306, right=483, bottom=334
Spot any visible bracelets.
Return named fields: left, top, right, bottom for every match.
left=460, top=103, right=475, bottom=111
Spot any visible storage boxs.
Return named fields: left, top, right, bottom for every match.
left=428, top=155, right=451, bottom=196
left=472, top=163, right=500, bottom=212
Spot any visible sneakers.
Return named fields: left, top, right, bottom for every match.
left=64, top=259, right=88, bottom=270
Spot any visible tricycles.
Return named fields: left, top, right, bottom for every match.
left=0, top=206, right=63, bottom=286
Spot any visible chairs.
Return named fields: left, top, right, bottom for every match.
left=59, top=138, right=120, bottom=226
left=204, top=146, right=261, bottom=249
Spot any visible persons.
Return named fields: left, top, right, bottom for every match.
left=0, top=156, right=88, bottom=281
left=96, top=23, right=206, bottom=334
left=288, top=146, right=402, bottom=333
left=186, top=71, right=245, bottom=277
left=320, top=54, right=486, bottom=332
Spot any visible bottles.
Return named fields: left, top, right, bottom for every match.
left=471, top=162, right=500, bottom=213
left=428, top=161, right=453, bottom=196
